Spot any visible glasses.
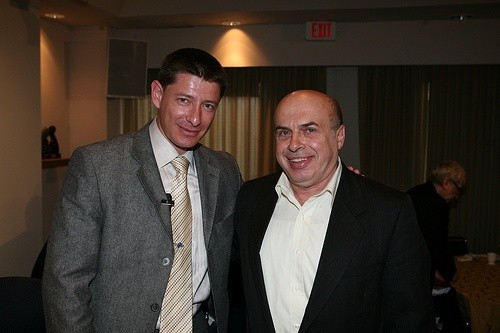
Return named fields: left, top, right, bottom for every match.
left=448, top=178, right=462, bottom=196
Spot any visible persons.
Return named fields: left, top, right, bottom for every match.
left=430, top=256, right=472, bottom=333
left=404, top=159, right=470, bottom=265
left=229, top=91, right=430, bottom=333
left=44, top=48, right=366, bottom=333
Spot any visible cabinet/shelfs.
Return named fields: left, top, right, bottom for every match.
left=40, top=158, right=69, bottom=169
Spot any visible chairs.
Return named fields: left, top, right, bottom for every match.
left=451, top=250, right=500, bottom=333
left=0, top=277, right=42, bottom=333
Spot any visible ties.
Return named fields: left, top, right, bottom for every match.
left=158, top=155, right=194, bottom=333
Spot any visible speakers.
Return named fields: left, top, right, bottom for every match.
left=105, top=37, right=149, bottom=99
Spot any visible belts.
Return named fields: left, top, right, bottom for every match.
left=190, top=299, right=210, bottom=318
